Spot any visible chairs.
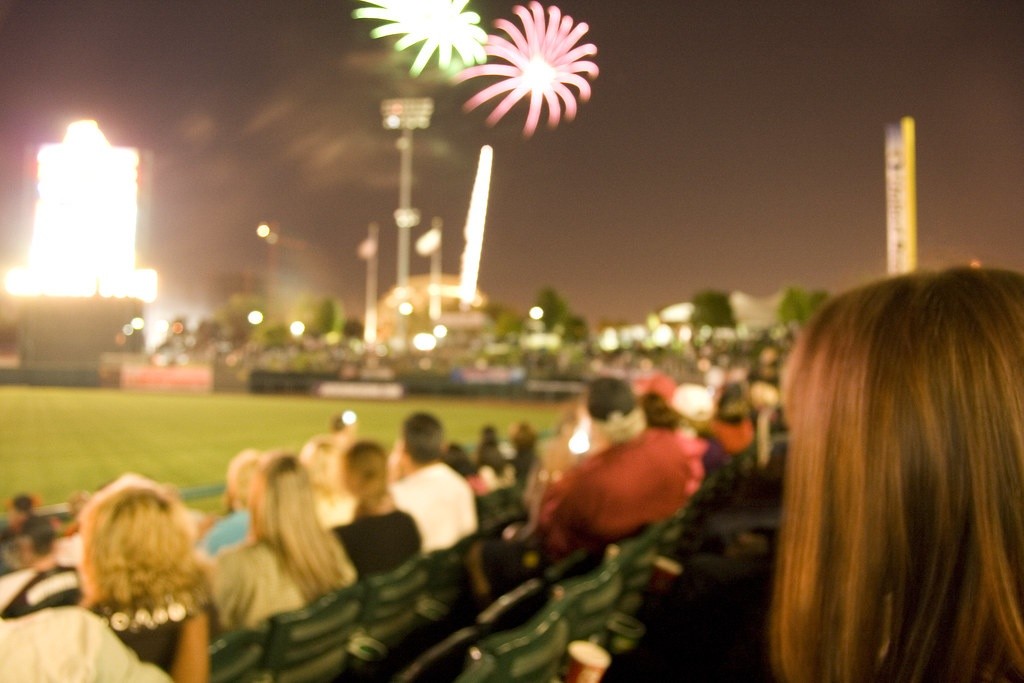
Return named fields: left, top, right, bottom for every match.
left=208, top=448, right=755, bottom=683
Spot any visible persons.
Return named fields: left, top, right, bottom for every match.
left=501, top=364, right=783, bottom=573
left=770, top=265, right=1024, bottom=683
left=0, top=410, right=537, bottom=683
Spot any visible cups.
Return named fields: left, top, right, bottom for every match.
left=562, top=639, right=611, bottom=683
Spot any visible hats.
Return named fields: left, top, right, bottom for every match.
left=588, top=375, right=634, bottom=415
